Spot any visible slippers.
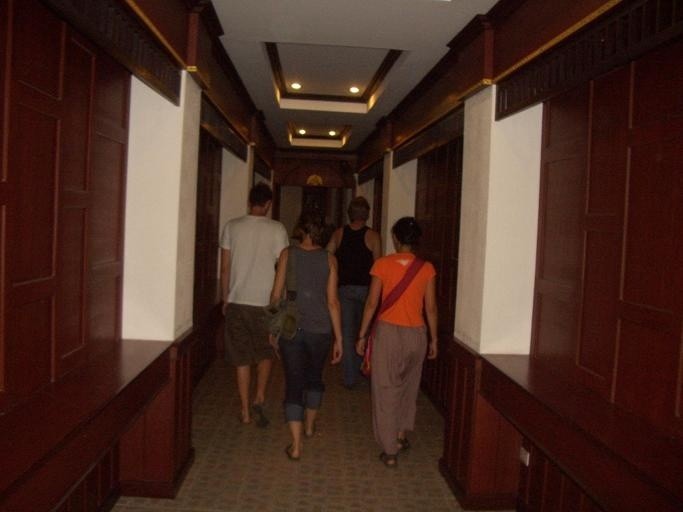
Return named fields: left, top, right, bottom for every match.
left=239, top=404, right=317, bottom=461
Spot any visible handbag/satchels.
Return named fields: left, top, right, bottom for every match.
left=360, top=257, right=425, bottom=377
left=262, top=247, right=301, bottom=343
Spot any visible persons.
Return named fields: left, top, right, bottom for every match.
left=353, top=216, right=437, bottom=468
left=219, top=181, right=289, bottom=424
left=322, top=196, right=382, bottom=387
left=265, top=210, right=343, bottom=459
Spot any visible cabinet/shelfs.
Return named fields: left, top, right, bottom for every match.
left=484, top=348, right=682, bottom=511
left=1, top=339, right=180, bottom=510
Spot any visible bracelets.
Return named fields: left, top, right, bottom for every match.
left=358, top=336, right=365, bottom=339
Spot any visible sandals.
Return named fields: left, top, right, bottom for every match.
left=396, top=438, right=411, bottom=454
left=378, top=451, right=398, bottom=467
